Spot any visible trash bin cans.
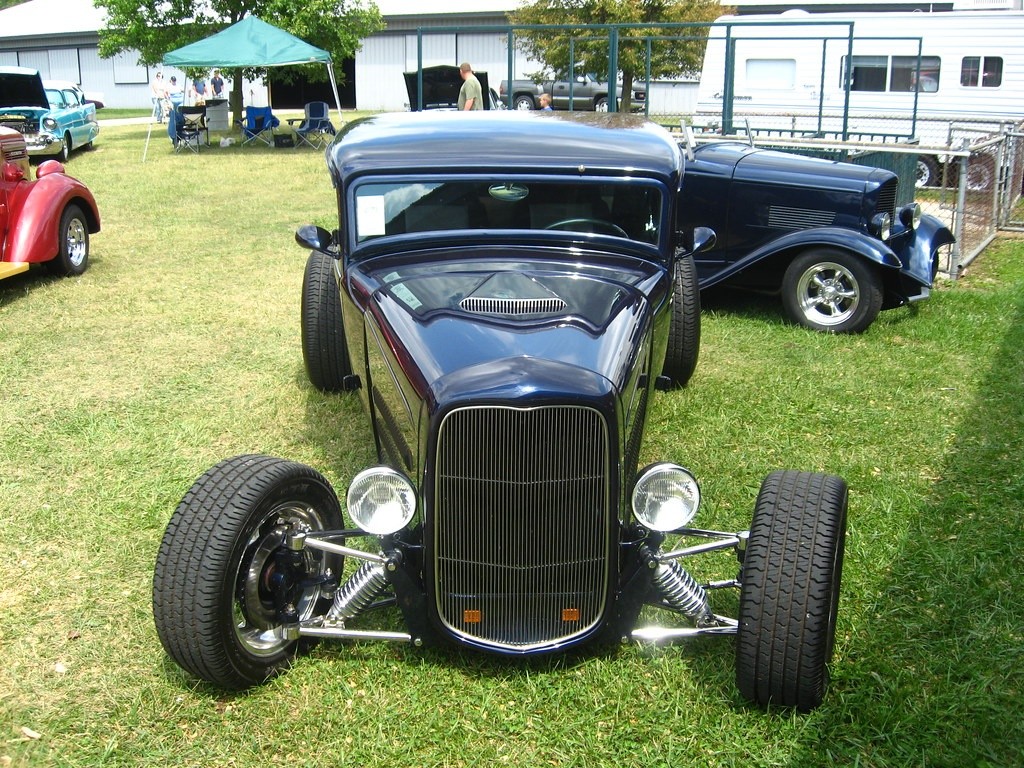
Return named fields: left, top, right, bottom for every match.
left=205, top=99, right=230, bottom=131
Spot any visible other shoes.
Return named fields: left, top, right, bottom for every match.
left=157, top=120, right=162, bottom=123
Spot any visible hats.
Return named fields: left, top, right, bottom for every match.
left=171, top=76, right=176, bottom=81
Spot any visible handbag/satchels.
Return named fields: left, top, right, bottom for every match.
left=274, top=133, right=294, bottom=147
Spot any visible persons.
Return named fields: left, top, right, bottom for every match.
left=539, top=93, right=553, bottom=112
left=459, top=62, right=483, bottom=111
left=151, top=69, right=225, bottom=124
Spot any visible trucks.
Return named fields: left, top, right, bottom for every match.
left=692, top=0, right=1023, bottom=194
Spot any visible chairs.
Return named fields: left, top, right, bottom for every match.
left=518, top=181, right=611, bottom=234
left=286, top=101, right=336, bottom=150
left=174, top=107, right=211, bottom=155
left=232, top=105, right=280, bottom=148
left=384, top=189, right=487, bottom=238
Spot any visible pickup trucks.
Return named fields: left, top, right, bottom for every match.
left=500, top=59, right=647, bottom=115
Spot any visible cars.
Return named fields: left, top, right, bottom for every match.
left=404, top=64, right=508, bottom=111
left=0, top=124, right=102, bottom=281
left=0, top=65, right=101, bottom=162
left=679, top=128, right=959, bottom=337
left=150, top=111, right=851, bottom=717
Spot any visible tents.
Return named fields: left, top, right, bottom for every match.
left=143, top=16, right=345, bottom=162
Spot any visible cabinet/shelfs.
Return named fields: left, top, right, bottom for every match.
left=205, top=99, right=229, bottom=131
